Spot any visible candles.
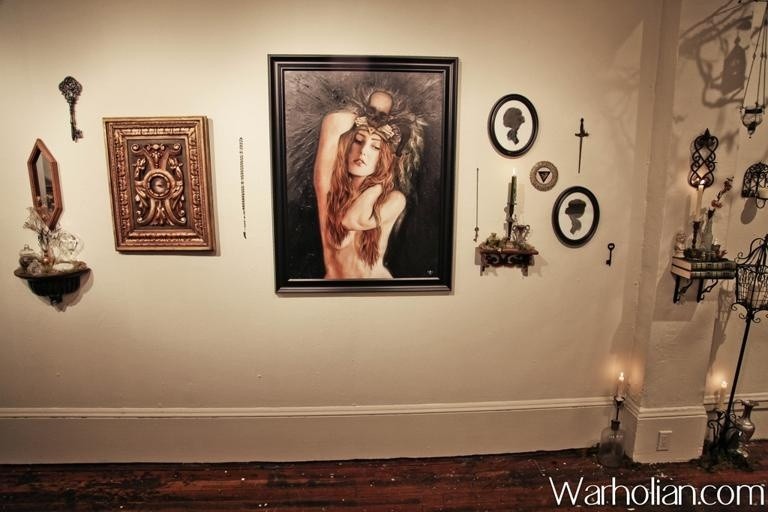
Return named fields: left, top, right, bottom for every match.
left=691, top=172, right=768, bottom=310
left=717, top=379, right=728, bottom=410
left=614, top=371, right=624, bottom=402
left=509, top=169, right=520, bottom=207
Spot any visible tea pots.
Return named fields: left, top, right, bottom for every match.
left=731, top=397, right=760, bottom=447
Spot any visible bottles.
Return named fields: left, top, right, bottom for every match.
left=596, top=420, right=626, bottom=467
left=698, top=216, right=715, bottom=253
left=19, top=243, right=35, bottom=272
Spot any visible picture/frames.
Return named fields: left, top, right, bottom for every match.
left=103, top=116, right=216, bottom=255
left=553, top=185, right=600, bottom=247
left=489, top=93, right=540, bottom=157
left=529, top=161, right=558, bottom=192
left=267, top=55, right=455, bottom=293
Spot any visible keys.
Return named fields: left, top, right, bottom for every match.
left=605, top=243, right=615, bottom=268
left=58, top=76, right=83, bottom=141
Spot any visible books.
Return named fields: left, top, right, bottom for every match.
left=670, top=264, right=736, bottom=280
left=671, top=256, right=736, bottom=271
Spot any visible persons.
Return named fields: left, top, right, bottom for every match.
left=311, top=108, right=407, bottom=278
